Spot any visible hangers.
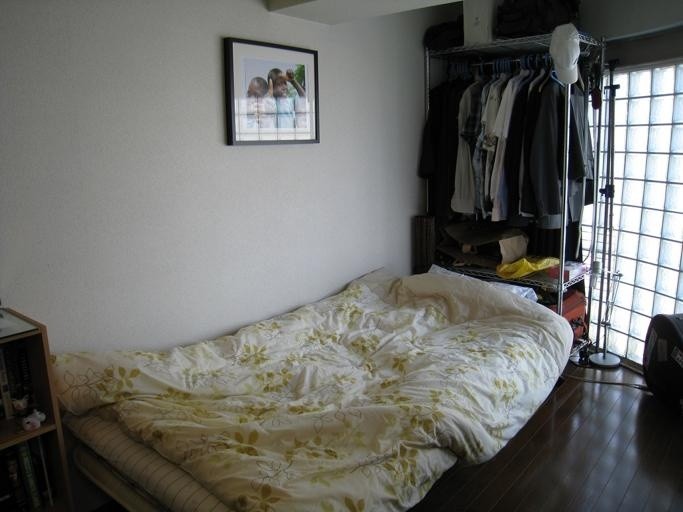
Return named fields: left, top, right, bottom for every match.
left=445, top=53, right=568, bottom=83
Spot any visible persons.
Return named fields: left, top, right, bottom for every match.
left=247, top=68, right=306, bottom=129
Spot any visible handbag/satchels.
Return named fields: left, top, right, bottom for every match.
left=438, top=223, right=528, bottom=268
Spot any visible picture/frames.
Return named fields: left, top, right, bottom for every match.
left=224, top=36, right=322, bottom=146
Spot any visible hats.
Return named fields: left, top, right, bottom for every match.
left=550, top=23, right=580, bottom=84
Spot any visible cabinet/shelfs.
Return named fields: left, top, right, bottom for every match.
left=0, top=305, right=74, bottom=509
left=424, top=25, right=608, bottom=368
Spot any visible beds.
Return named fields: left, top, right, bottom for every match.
left=49, top=265, right=577, bottom=511
